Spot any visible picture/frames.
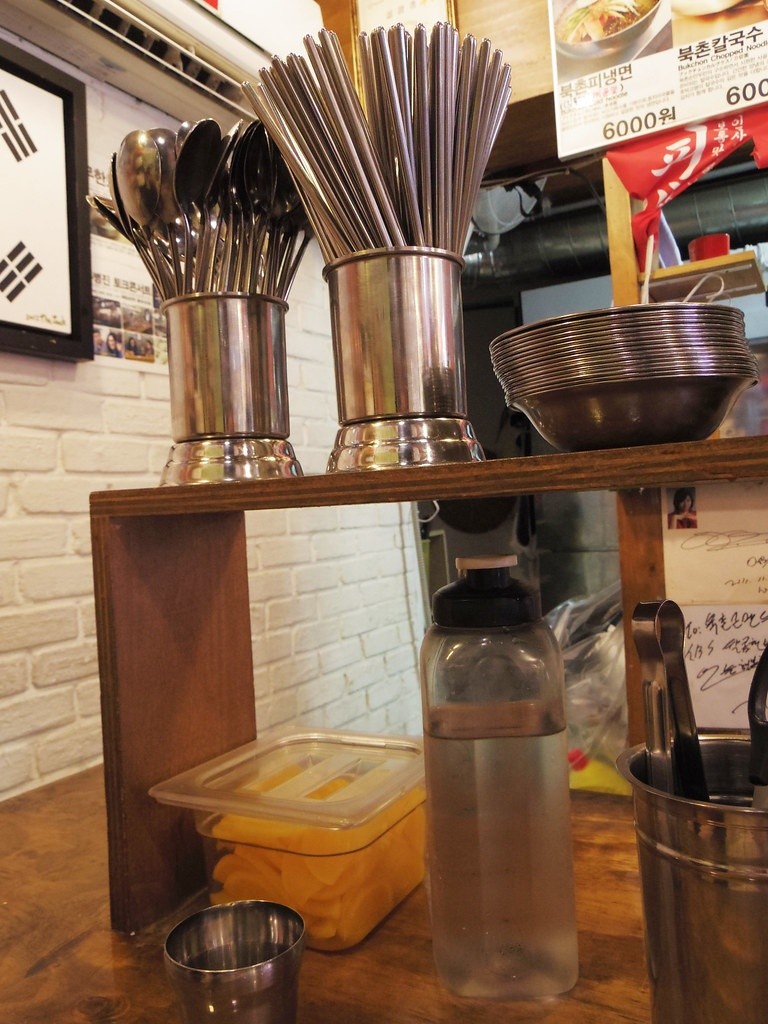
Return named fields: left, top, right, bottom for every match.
left=0, top=39, right=95, bottom=364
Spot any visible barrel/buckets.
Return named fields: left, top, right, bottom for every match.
left=616, top=732, right=767, bottom=1024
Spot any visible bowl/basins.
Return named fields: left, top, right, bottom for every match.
left=555, top=0, right=662, bottom=58
left=486, top=300, right=762, bottom=451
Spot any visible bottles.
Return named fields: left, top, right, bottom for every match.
left=418, top=557, right=579, bottom=999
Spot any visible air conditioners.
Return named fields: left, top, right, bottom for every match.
left=0, top=0, right=273, bottom=135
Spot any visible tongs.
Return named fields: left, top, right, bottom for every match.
left=630, top=599, right=710, bottom=802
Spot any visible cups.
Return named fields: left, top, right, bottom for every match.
left=163, top=898, right=305, bottom=1024
left=159, top=291, right=290, bottom=442
left=321, top=246, right=468, bottom=427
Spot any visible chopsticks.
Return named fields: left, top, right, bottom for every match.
left=239, top=20, right=513, bottom=267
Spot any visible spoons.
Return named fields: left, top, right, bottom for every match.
left=86, top=117, right=313, bottom=300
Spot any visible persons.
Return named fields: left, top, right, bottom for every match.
left=125, top=337, right=141, bottom=355
left=667, top=488, right=697, bottom=529
left=106, top=334, right=123, bottom=358
left=93, top=328, right=109, bottom=354
left=141, top=340, right=153, bottom=355
